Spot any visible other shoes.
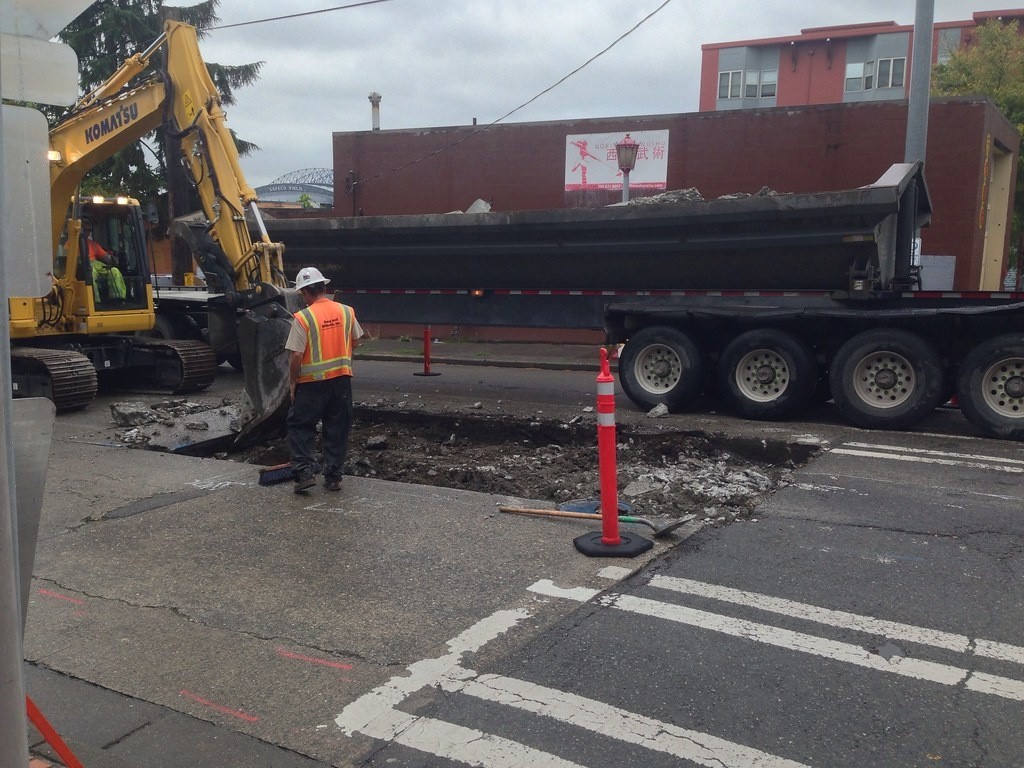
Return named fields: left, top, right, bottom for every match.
left=294, top=467, right=317, bottom=492
left=109, top=298, right=126, bottom=306
left=325, top=471, right=342, bottom=490
left=94, top=302, right=107, bottom=309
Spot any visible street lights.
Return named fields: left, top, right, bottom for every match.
left=616, top=133, right=643, bottom=203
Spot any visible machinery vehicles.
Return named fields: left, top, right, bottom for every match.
left=10, top=19, right=337, bottom=445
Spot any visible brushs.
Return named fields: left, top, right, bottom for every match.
left=260, top=461, right=321, bottom=485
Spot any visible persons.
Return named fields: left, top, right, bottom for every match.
left=285, top=267, right=364, bottom=493
left=63, top=220, right=127, bottom=309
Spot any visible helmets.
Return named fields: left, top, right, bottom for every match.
left=293, top=267, right=331, bottom=292
